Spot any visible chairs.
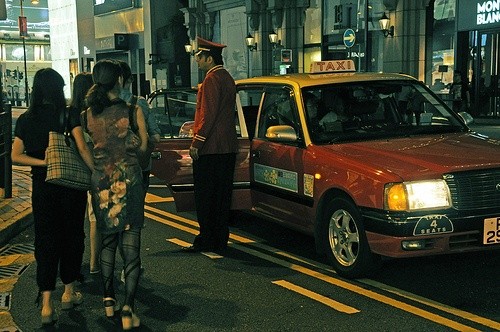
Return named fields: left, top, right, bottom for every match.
left=352, top=101, right=381, bottom=125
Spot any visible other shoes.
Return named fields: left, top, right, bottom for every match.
left=183, top=245, right=208, bottom=253
left=90, top=269, right=101, bottom=274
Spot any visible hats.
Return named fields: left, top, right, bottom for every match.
left=190, top=37, right=227, bottom=56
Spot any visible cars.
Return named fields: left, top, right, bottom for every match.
left=134, top=60, right=500, bottom=281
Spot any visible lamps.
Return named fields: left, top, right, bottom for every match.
left=269, top=30, right=281, bottom=48
left=245, top=33, right=258, bottom=52
left=184, top=41, right=195, bottom=56
left=379, top=12, right=394, bottom=38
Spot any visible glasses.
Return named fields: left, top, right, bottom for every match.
left=305, top=104, right=319, bottom=109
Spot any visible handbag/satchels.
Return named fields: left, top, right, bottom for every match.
left=44, top=106, right=95, bottom=190
left=125, top=104, right=155, bottom=158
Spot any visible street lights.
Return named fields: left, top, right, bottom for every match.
left=18, top=0, right=40, bottom=108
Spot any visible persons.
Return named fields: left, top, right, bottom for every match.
left=448, top=70, right=470, bottom=114
left=317, top=93, right=352, bottom=134
left=69, top=58, right=160, bottom=331
left=10, top=69, right=99, bottom=324
left=180, top=36, right=238, bottom=251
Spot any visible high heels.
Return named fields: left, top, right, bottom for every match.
left=61, top=291, right=83, bottom=310
left=103, top=297, right=121, bottom=317
left=41, top=306, right=58, bottom=323
left=122, top=305, right=140, bottom=330
